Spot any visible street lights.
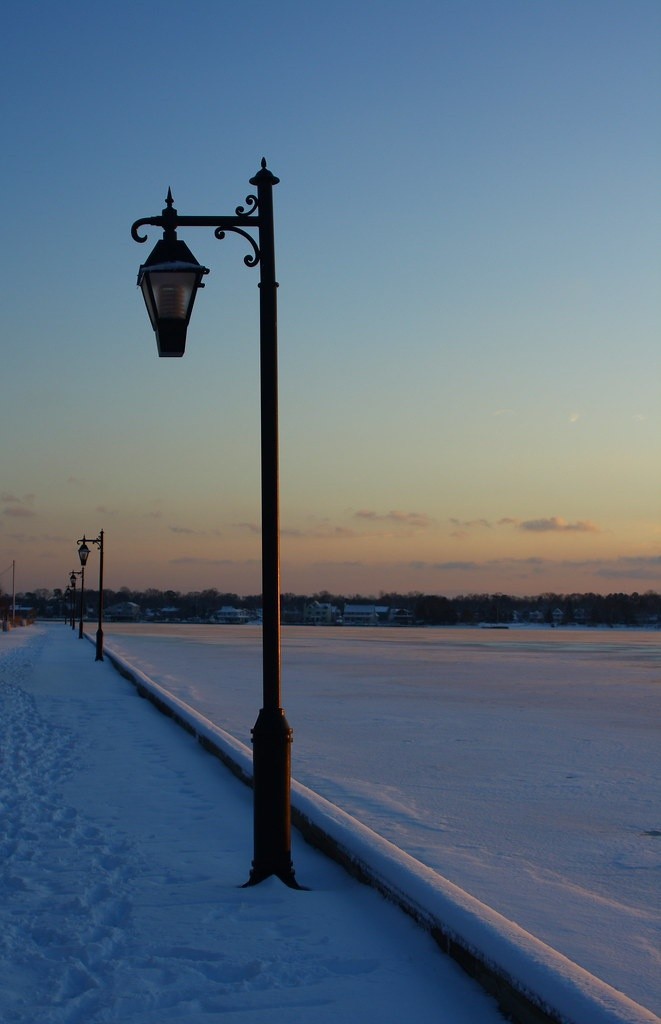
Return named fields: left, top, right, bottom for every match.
left=77, top=528, right=105, bottom=661
left=132, top=155, right=311, bottom=891
left=70, top=566, right=85, bottom=640
left=63, top=585, right=74, bottom=627
